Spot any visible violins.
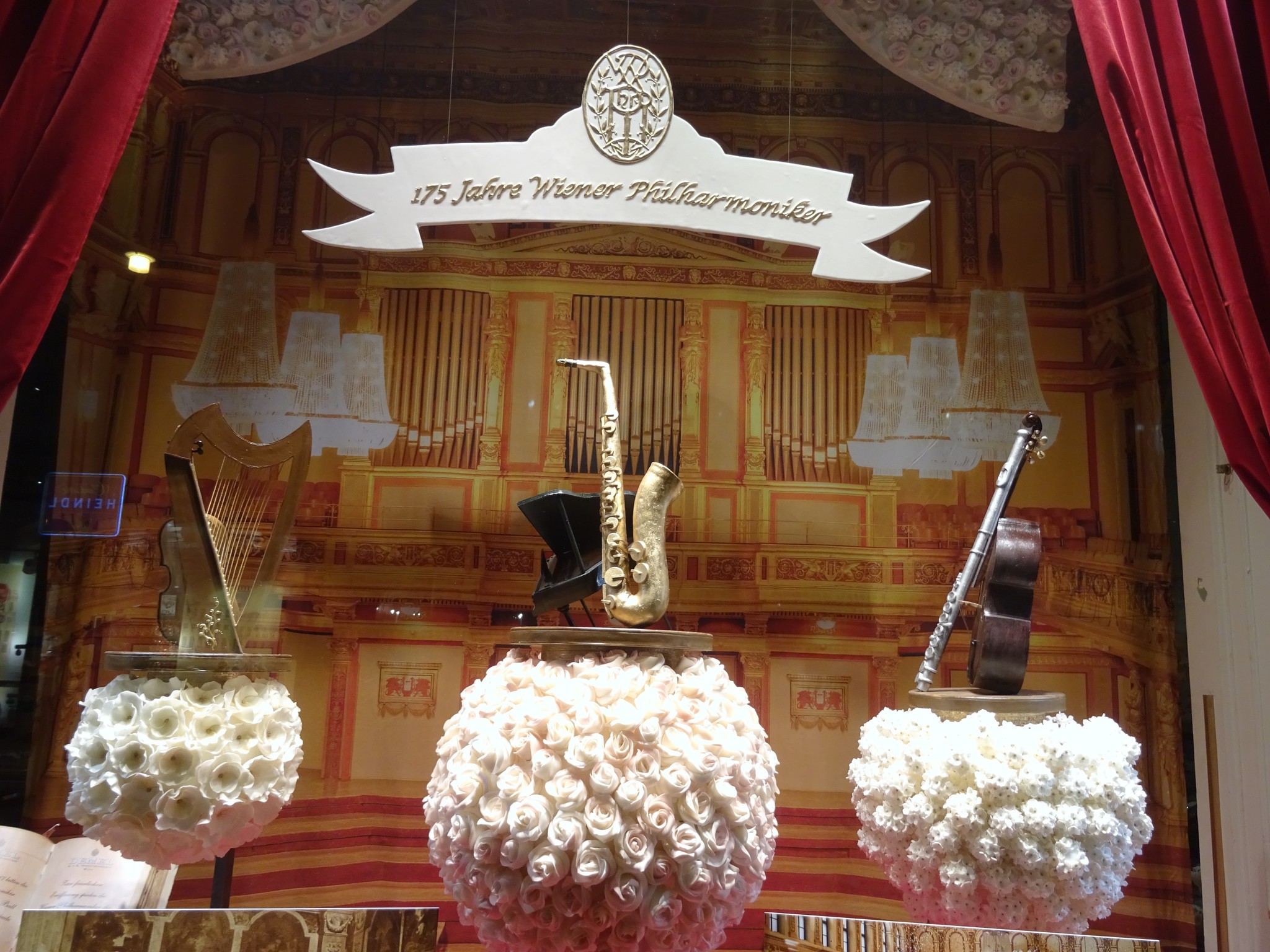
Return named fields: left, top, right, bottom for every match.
left=157, top=424, right=241, bottom=649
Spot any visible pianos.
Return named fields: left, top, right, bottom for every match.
left=517, top=489, right=674, bottom=630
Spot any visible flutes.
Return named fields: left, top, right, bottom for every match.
left=914, top=429, right=1031, bottom=694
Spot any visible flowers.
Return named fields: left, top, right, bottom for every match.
left=423, top=647, right=779, bottom=945
left=847, top=709, right=1157, bottom=928
left=60, top=675, right=304, bottom=869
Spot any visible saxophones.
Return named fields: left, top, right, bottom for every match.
left=555, top=357, right=686, bottom=628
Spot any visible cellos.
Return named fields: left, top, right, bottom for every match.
left=966, top=411, right=1048, bottom=695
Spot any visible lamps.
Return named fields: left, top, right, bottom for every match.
left=124, top=248, right=159, bottom=275
